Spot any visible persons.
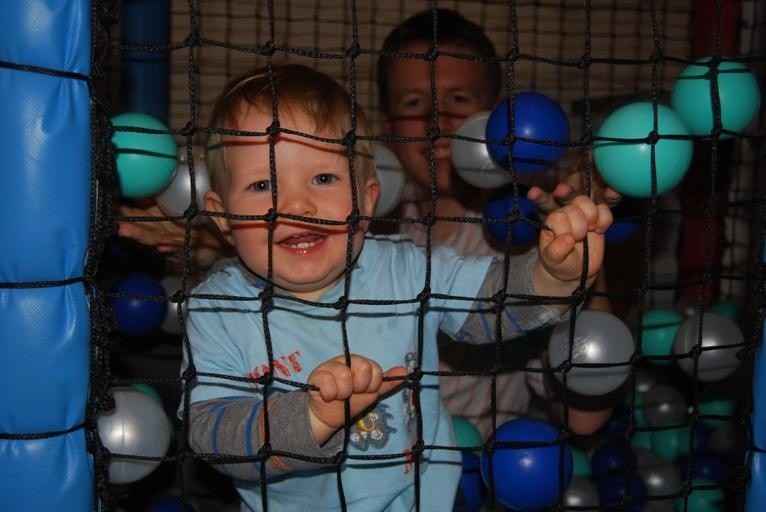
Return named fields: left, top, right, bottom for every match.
left=95, top=7, right=765, bottom=511
left=173, top=64, right=618, bottom=511
left=113, top=7, right=620, bottom=449
